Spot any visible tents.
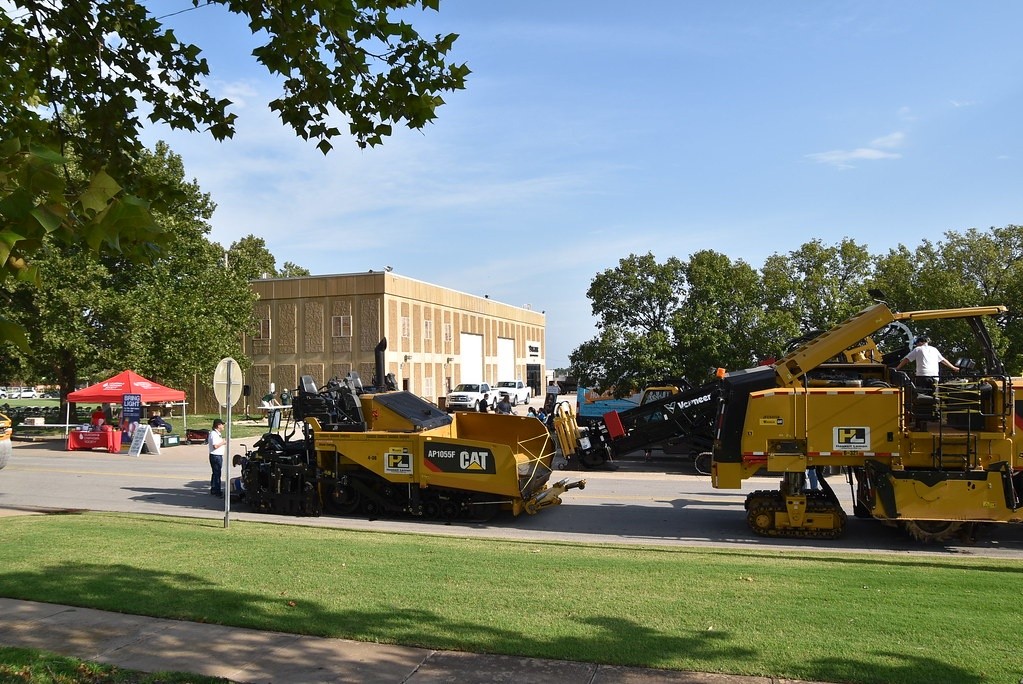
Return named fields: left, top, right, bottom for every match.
left=65, top=368, right=187, bottom=436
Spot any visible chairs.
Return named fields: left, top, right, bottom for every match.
left=465, top=385, right=473, bottom=391
left=36, top=407, right=49, bottom=418
left=76, top=406, right=84, bottom=418
left=349, top=372, right=364, bottom=395
left=299, top=375, right=333, bottom=416
left=17, top=407, right=32, bottom=419
left=48, top=406, right=60, bottom=418
left=30, top=407, right=38, bottom=416
left=82, top=407, right=91, bottom=419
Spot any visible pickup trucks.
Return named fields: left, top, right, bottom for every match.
left=446, top=383, right=501, bottom=413
left=494, top=380, right=531, bottom=407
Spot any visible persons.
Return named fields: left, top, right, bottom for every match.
left=893, top=336, right=961, bottom=428
left=261, top=390, right=280, bottom=407
left=90, top=406, right=107, bottom=426
left=537, top=408, right=547, bottom=422
left=526, top=407, right=537, bottom=417
left=281, top=388, right=292, bottom=405
left=208, top=418, right=226, bottom=498
left=478, top=394, right=489, bottom=413
left=496, top=394, right=517, bottom=416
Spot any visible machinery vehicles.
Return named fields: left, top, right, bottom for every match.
left=232, top=337, right=589, bottom=524
left=555, top=304, right=1023, bottom=543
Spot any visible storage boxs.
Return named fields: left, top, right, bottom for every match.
left=160, top=434, right=181, bottom=446
left=25, top=418, right=44, bottom=425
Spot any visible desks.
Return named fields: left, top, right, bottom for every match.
left=17, top=423, right=83, bottom=446
left=152, top=426, right=166, bottom=434
left=67, top=430, right=121, bottom=453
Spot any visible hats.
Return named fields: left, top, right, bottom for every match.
left=284, top=389, right=287, bottom=391
left=214, top=419, right=225, bottom=424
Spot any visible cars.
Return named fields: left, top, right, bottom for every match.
left=40, top=390, right=61, bottom=399
left=8, top=389, right=38, bottom=398
left=0, top=389, right=7, bottom=399
